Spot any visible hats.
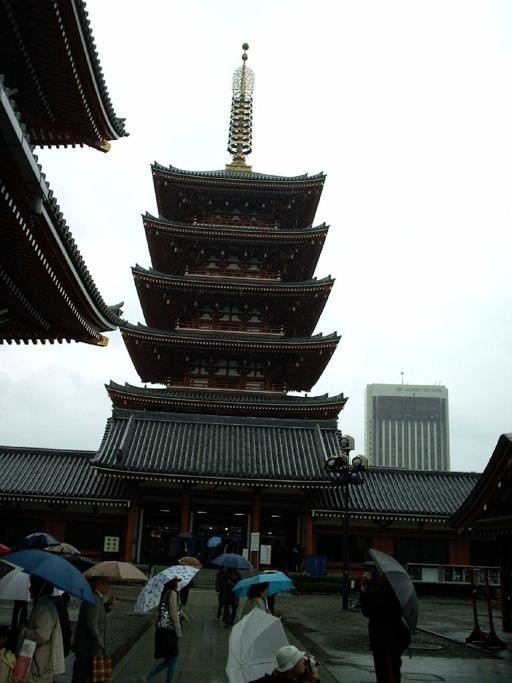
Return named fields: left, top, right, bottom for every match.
left=276, top=645, right=306, bottom=672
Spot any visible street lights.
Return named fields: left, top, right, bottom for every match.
left=324, top=432, right=369, bottom=613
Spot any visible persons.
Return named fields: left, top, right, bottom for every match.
left=1, top=533, right=420, bottom=683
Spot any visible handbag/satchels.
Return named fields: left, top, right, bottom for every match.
left=154, top=627, right=179, bottom=658
left=92, top=656, right=112, bottom=683
